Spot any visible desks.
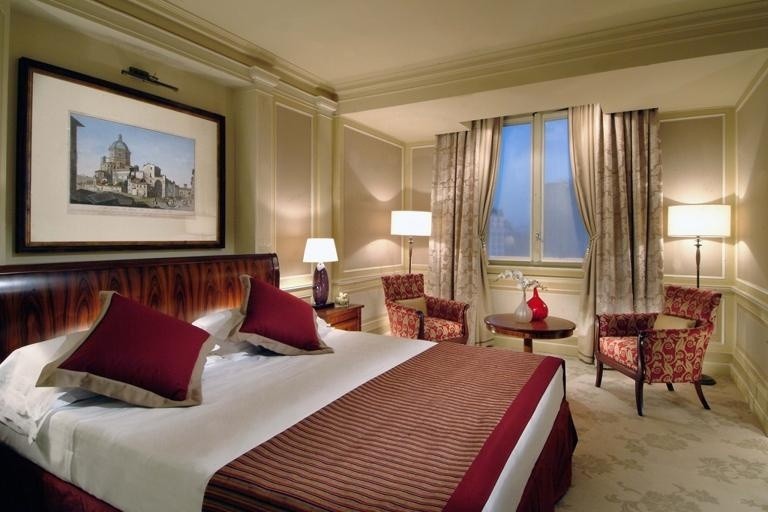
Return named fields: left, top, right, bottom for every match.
left=484, top=314, right=576, bottom=354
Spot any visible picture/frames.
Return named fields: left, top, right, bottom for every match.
left=16, top=57, right=229, bottom=252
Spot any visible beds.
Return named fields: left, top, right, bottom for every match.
left=1, top=250, right=578, bottom=511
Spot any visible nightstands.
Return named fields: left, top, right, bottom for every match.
left=308, top=299, right=365, bottom=333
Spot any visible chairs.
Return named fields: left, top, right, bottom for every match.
left=379, top=274, right=473, bottom=346
left=594, top=285, right=723, bottom=415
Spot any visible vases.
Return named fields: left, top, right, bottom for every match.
left=513, top=290, right=533, bottom=322
left=528, top=291, right=551, bottom=321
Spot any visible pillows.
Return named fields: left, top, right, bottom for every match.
left=652, top=314, right=695, bottom=333
left=36, top=290, right=216, bottom=409
left=190, top=307, right=256, bottom=356
left=2, top=333, right=113, bottom=444
left=228, top=274, right=335, bottom=356
left=396, top=296, right=428, bottom=316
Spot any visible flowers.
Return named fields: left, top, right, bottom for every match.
left=495, top=268, right=540, bottom=291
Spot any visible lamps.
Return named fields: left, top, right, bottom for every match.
left=301, top=237, right=339, bottom=307
left=390, top=209, right=433, bottom=274
left=665, top=205, right=732, bottom=387
